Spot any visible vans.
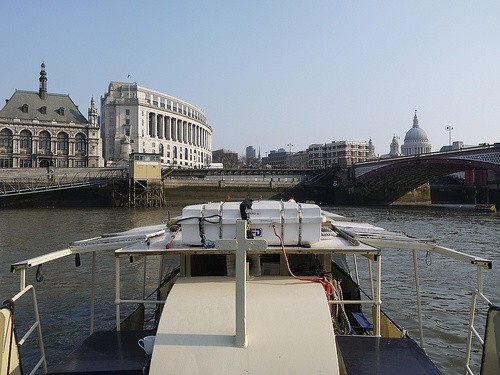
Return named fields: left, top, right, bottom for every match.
left=205, top=162, right=223, bottom=169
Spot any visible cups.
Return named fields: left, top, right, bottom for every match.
left=138, top=336, right=156, bottom=355
